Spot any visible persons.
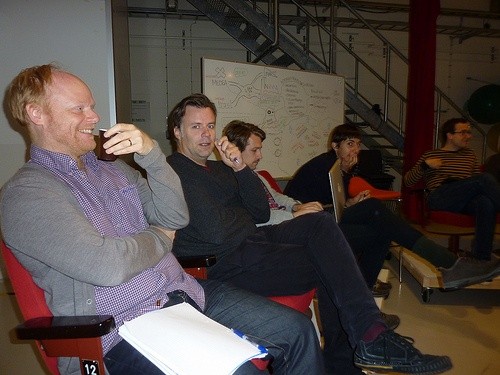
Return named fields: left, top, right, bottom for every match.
left=284, top=124, right=392, bottom=298
left=166, top=93, right=454, bottom=375
left=406, top=116, right=499, bottom=260
left=222, top=119, right=499, bottom=332
left=1, top=65, right=324, bottom=374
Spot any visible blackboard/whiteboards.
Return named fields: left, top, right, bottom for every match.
left=200, top=56, right=345, bottom=180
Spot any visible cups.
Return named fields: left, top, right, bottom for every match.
left=97, top=128, right=121, bottom=162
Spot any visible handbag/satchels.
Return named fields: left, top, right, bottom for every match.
left=437, top=177, right=473, bottom=205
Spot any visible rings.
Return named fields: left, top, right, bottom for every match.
left=128, top=139, right=133, bottom=147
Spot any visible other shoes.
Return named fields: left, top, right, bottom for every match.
left=371, top=282, right=390, bottom=297
left=377, top=278, right=392, bottom=289
left=380, top=309, right=400, bottom=330
left=437, top=249, right=500, bottom=292
left=353, top=329, right=452, bottom=374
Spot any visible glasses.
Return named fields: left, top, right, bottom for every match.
left=454, top=130, right=472, bottom=135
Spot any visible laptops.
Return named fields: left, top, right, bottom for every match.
left=329, top=159, right=345, bottom=224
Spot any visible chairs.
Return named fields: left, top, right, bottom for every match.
left=0, top=171, right=500, bottom=375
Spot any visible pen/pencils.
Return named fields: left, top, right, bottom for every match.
left=215, top=138, right=240, bottom=165
left=231, top=328, right=268, bottom=353
